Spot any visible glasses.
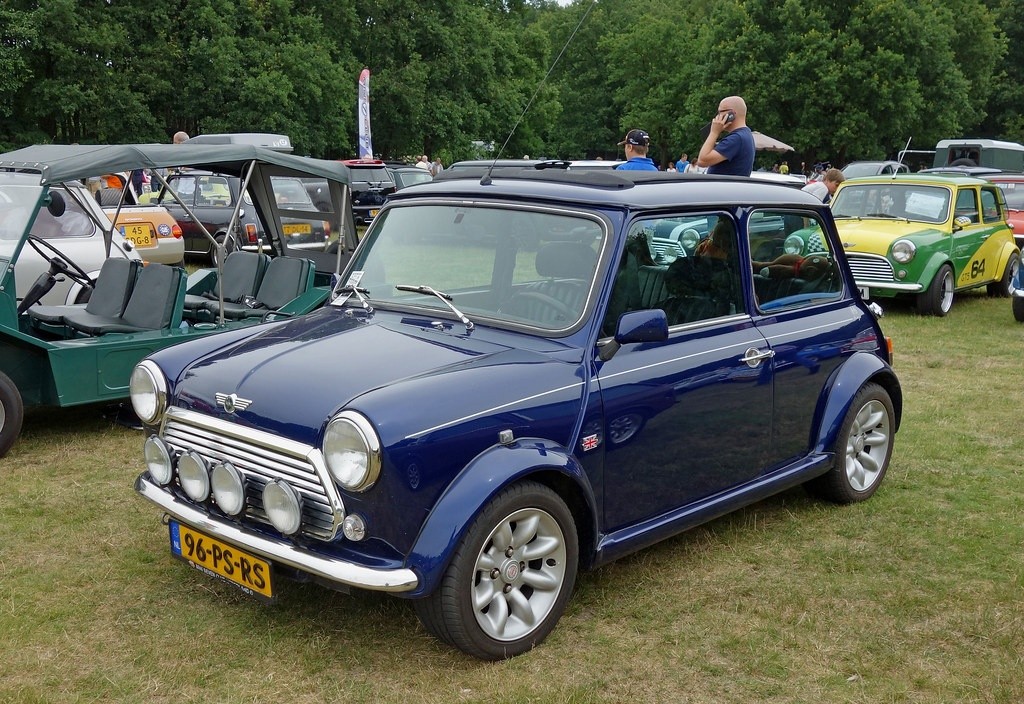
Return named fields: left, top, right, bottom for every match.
left=718, top=109, right=731, bottom=114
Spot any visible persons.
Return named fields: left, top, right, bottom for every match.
left=772, top=161, right=789, bottom=174
left=697, top=96, right=755, bottom=177
left=173, top=131, right=202, bottom=200
left=800, top=162, right=810, bottom=177
left=801, top=168, right=846, bottom=202
left=131, top=169, right=143, bottom=197
left=150, top=169, right=164, bottom=192
left=666, top=162, right=676, bottom=172
left=614, top=128, right=658, bottom=172
left=684, top=158, right=698, bottom=174
left=675, top=153, right=690, bottom=173
left=415, top=155, right=443, bottom=177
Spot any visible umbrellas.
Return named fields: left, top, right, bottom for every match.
left=751, top=130, right=795, bottom=153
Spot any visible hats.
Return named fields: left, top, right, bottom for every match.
left=617, top=129, right=649, bottom=147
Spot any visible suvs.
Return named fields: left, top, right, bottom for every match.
left=130, top=161, right=904, bottom=665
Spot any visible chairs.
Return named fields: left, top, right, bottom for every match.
left=184, top=251, right=272, bottom=319
left=96, top=188, right=125, bottom=207
left=27, top=258, right=144, bottom=329
left=204, top=256, right=316, bottom=324
left=513, top=241, right=612, bottom=330
left=63, top=263, right=188, bottom=334
left=649, top=256, right=737, bottom=326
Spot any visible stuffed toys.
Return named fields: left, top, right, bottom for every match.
left=751, top=253, right=831, bottom=281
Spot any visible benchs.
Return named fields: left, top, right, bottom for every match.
left=629, top=264, right=817, bottom=304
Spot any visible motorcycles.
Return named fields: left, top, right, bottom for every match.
left=0, top=144, right=363, bottom=458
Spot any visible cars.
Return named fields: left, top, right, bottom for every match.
left=1, top=138, right=1024, bottom=313
left=782, top=174, right=1024, bottom=318
left=1008, top=244, right=1024, bottom=324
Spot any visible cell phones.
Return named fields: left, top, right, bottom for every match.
left=722, top=111, right=736, bottom=125
